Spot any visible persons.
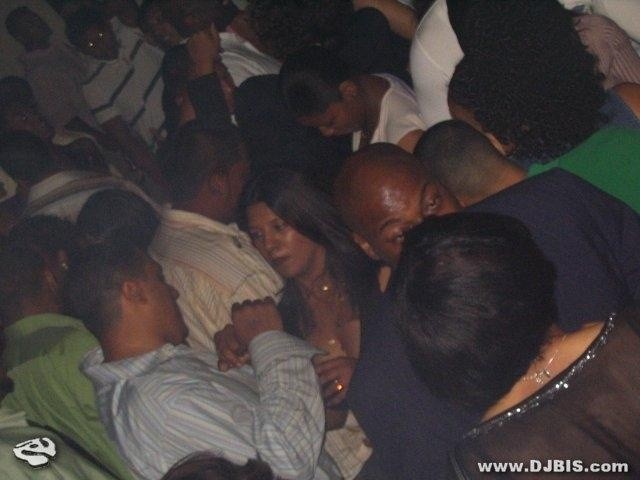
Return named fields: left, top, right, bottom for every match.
left=0, top=0, right=640, bottom=480
